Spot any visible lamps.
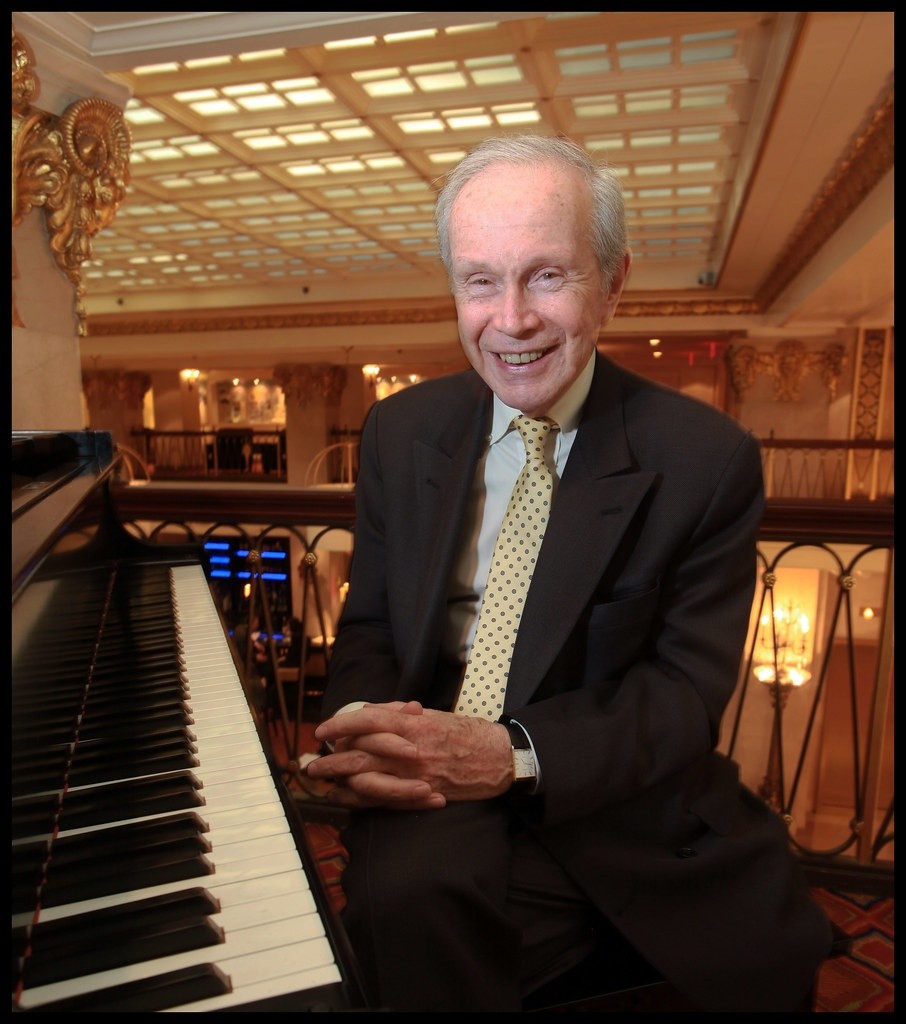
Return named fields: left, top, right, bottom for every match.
left=756, top=601, right=812, bottom=815
left=180, top=368, right=200, bottom=390
left=363, top=365, right=380, bottom=386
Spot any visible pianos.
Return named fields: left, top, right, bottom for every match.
left=12, top=558, right=372, bottom=1013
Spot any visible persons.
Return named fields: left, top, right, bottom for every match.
left=302, top=135, right=824, bottom=1019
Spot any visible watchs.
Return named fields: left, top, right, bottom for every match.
left=498, top=719, right=537, bottom=796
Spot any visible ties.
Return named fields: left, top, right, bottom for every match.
left=451, top=413, right=557, bottom=720
